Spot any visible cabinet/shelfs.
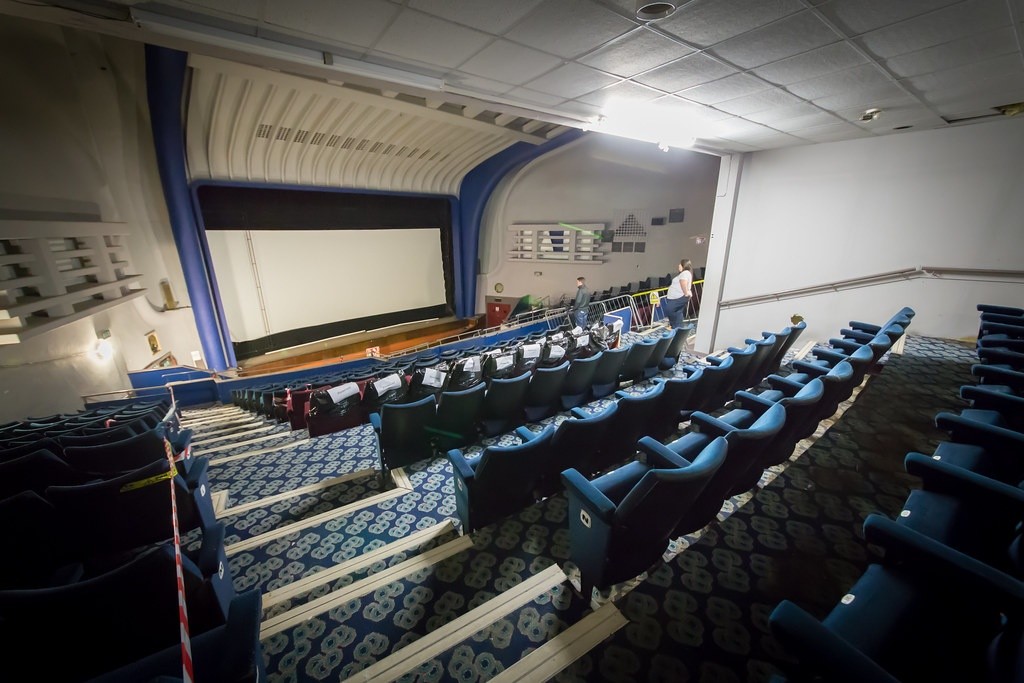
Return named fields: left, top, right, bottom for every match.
left=0, top=220, right=149, bottom=346
left=134, top=351, right=178, bottom=372
left=507, top=223, right=605, bottom=265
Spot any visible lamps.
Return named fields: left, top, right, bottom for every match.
left=131, top=7, right=446, bottom=93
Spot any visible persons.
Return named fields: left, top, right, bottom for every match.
left=573, top=277, right=591, bottom=326
left=654, top=259, right=693, bottom=329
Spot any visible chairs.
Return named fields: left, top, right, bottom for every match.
left=0, top=260, right=1024, bottom=682
